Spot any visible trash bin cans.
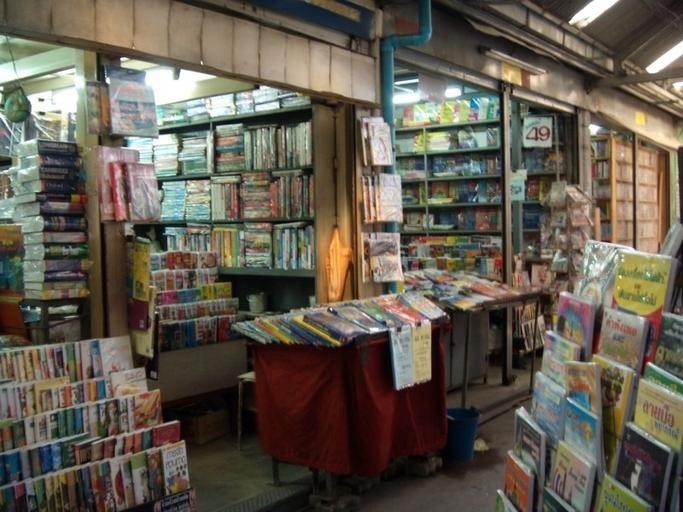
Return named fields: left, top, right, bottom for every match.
left=440, top=408, right=479, bottom=469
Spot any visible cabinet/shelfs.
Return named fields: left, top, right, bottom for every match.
left=524, top=139, right=574, bottom=267
left=397, top=117, right=502, bottom=241
left=592, top=133, right=661, bottom=253
left=138, top=105, right=316, bottom=283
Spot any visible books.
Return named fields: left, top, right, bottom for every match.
left=491, top=237, right=682, bottom=512
left=1, top=83, right=610, bottom=348
left=0, top=333, right=202, bottom=511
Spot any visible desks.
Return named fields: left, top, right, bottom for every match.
left=246, top=317, right=455, bottom=493
